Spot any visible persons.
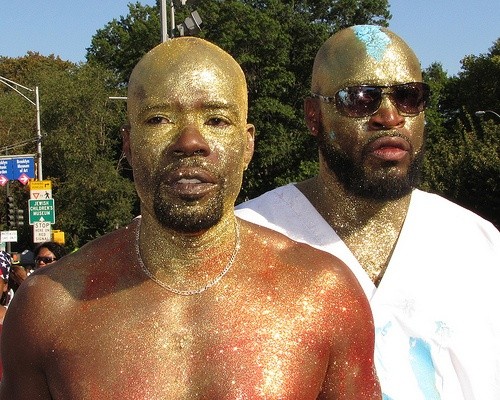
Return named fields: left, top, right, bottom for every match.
left=0, top=242, right=66, bottom=383
left=232, top=25, right=500, bottom=400
left=0, top=37, right=382, bottom=400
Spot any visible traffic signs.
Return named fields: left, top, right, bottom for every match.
left=28, top=198, right=56, bottom=225
left=0, top=155, right=35, bottom=187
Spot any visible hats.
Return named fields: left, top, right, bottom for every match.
left=0, top=250, right=11, bottom=282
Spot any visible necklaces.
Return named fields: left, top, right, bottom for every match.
left=136, top=218, right=240, bottom=295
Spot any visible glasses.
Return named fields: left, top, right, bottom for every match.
left=34, top=256, right=57, bottom=266
left=307, top=80, right=431, bottom=119
